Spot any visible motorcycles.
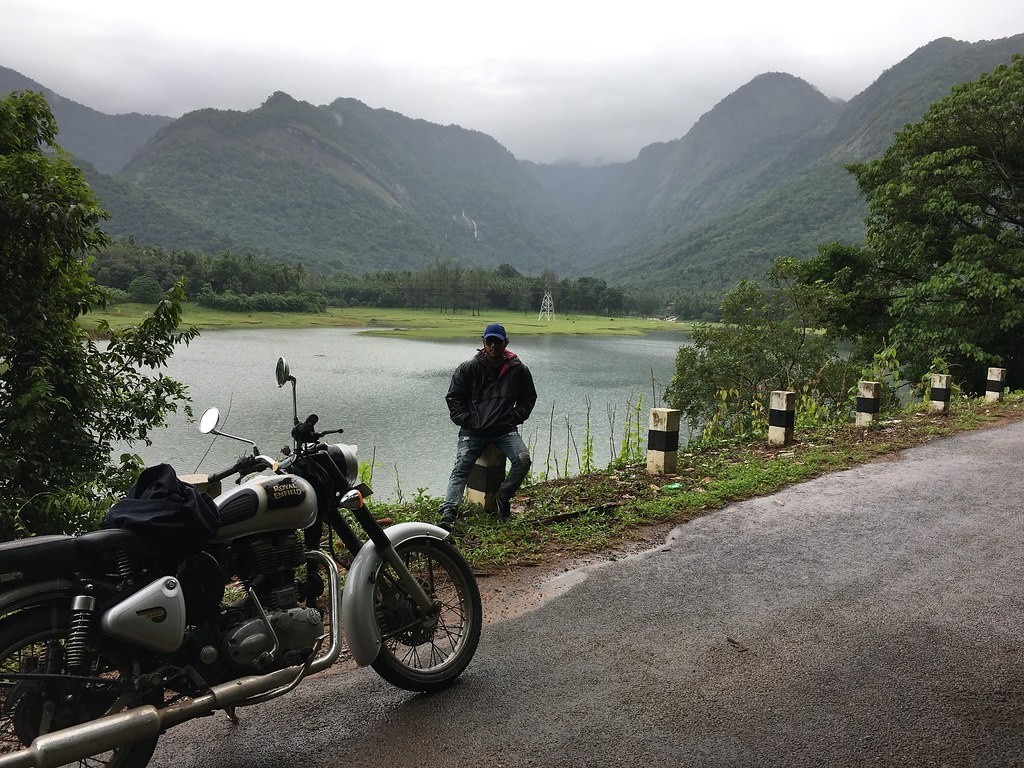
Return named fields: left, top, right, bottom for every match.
left=1, top=358, right=480, bottom=768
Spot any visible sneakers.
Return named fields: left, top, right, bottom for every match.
left=438, top=508, right=458, bottom=530
left=496, top=494, right=510, bottom=520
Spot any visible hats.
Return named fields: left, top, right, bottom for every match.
left=485, top=324, right=506, bottom=341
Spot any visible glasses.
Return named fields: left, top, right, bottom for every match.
left=483, top=339, right=504, bottom=346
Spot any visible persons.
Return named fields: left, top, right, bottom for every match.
left=438, top=324, right=537, bottom=533
